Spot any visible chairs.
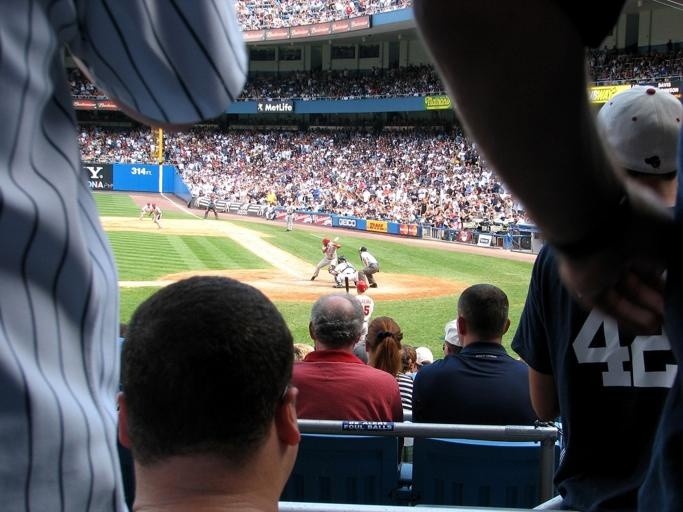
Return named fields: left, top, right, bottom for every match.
left=279, top=431, right=402, bottom=505
left=411, top=435, right=559, bottom=509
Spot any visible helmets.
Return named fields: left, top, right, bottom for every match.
left=359, top=246, right=367, bottom=251
left=338, top=256, right=347, bottom=264
left=355, top=281, right=368, bottom=292
left=322, top=238, right=331, bottom=246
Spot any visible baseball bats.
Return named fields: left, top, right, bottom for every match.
left=331, top=237, right=340, bottom=243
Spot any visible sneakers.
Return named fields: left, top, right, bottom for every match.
left=370, top=283, right=377, bottom=288
left=311, top=274, right=318, bottom=281
left=335, top=283, right=343, bottom=288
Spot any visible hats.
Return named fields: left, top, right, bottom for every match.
left=415, top=347, right=433, bottom=366
left=596, top=84, right=683, bottom=178
left=445, top=317, right=462, bottom=347
